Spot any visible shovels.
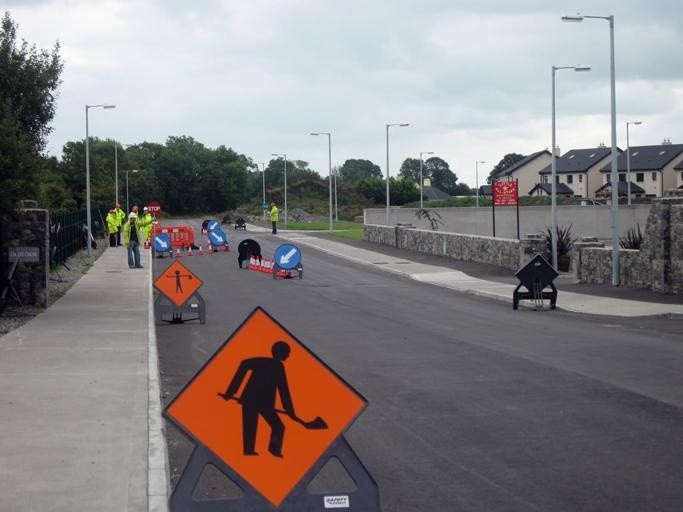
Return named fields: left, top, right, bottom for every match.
left=218, top=393, right=328, bottom=429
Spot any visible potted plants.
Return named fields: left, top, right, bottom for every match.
left=539, top=222, right=579, bottom=273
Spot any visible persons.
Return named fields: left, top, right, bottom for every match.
left=267, top=202, right=279, bottom=234
left=105, top=203, right=155, bottom=268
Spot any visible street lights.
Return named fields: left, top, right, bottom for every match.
left=560, top=14, right=622, bottom=288
left=308, top=131, right=334, bottom=230
left=549, top=63, right=592, bottom=273
left=384, top=121, right=408, bottom=227
left=475, top=160, right=485, bottom=209
left=418, top=150, right=434, bottom=209
left=82, top=104, right=114, bottom=258
left=253, top=161, right=266, bottom=221
left=270, top=152, right=288, bottom=228
left=124, top=168, right=137, bottom=218
left=625, top=120, right=644, bottom=209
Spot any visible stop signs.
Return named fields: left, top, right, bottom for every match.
left=147, top=202, right=160, bottom=215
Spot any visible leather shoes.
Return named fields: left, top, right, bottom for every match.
left=135, top=265, right=143, bottom=268
left=129, top=265, right=134, bottom=268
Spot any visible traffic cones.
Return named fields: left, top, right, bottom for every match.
left=205, top=242, right=214, bottom=254
left=174, top=247, right=183, bottom=257
left=196, top=242, right=204, bottom=255
left=185, top=244, right=193, bottom=256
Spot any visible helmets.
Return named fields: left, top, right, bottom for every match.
left=128, top=212, right=137, bottom=218
left=143, top=207, right=148, bottom=210
